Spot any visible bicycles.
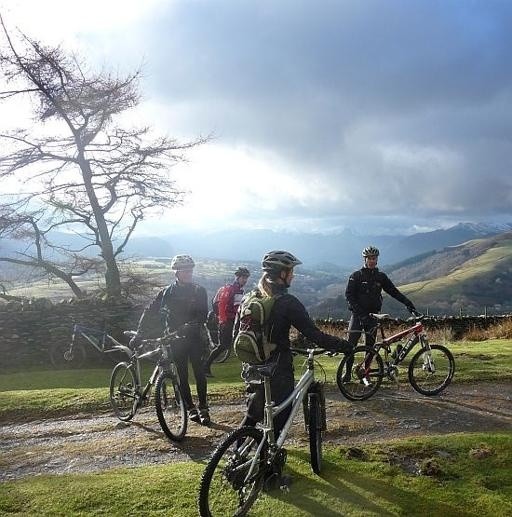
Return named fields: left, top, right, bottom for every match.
left=196, top=343, right=348, bottom=516
left=334, top=309, right=458, bottom=403
left=46, top=313, right=230, bottom=442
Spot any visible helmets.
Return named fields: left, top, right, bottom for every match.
left=171, top=255, right=195, bottom=269
left=262, top=250, right=303, bottom=271
left=235, top=267, right=250, bottom=277
left=362, top=246, right=379, bottom=257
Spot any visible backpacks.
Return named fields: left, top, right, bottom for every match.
left=233, top=289, right=289, bottom=364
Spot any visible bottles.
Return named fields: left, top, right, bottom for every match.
left=390, top=343, right=402, bottom=358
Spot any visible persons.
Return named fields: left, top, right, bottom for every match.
left=341, top=245, right=419, bottom=386
left=205, top=265, right=251, bottom=378
left=127, top=253, right=209, bottom=424
left=237, top=248, right=355, bottom=493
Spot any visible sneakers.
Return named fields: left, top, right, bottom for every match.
left=202, top=360, right=215, bottom=378
left=361, top=377, right=373, bottom=387
left=341, top=372, right=351, bottom=381
left=189, top=404, right=210, bottom=426
